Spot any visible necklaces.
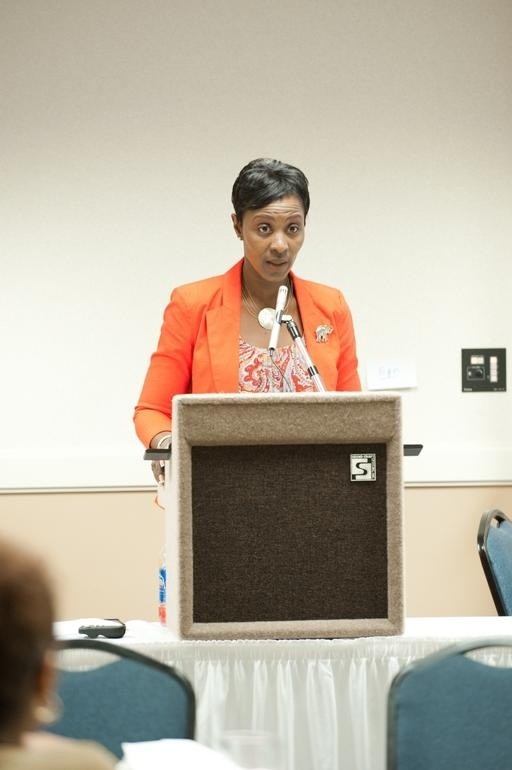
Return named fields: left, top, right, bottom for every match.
left=241, top=276, right=293, bottom=321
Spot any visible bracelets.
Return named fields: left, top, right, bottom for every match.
left=155, top=431, right=172, bottom=449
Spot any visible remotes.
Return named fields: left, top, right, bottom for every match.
left=78, top=625, right=126, bottom=638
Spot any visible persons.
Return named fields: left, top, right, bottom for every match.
left=1, top=533, right=125, bottom=768
left=129, top=156, right=363, bottom=488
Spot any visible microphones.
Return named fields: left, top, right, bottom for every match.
left=268, top=285, right=288, bottom=350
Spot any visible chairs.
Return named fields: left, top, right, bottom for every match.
left=29, top=636, right=195, bottom=765
left=475, top=506, right=510, bottom=616
left=382, top=632, right=511, bottom=770
